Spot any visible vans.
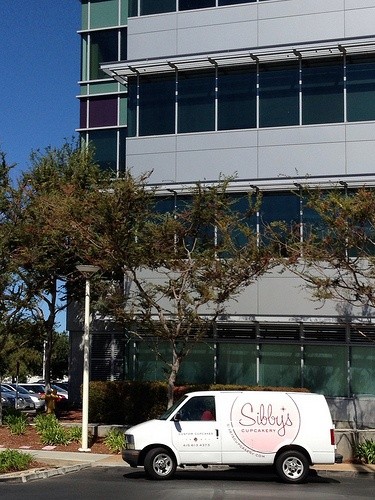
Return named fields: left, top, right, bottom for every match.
left=123, top=390, right=344, bottom=487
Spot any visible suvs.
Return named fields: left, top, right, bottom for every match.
left=0, top=384, right=34, bottom=415
left=11, top=384, right=47, bottom=413
left=29, top=381, right=70, bottom=406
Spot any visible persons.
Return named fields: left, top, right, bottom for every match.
left=198, top=400, right=213, bottom=421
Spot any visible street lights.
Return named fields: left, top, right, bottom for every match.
left=76, top=265, right=100, bottom=452
